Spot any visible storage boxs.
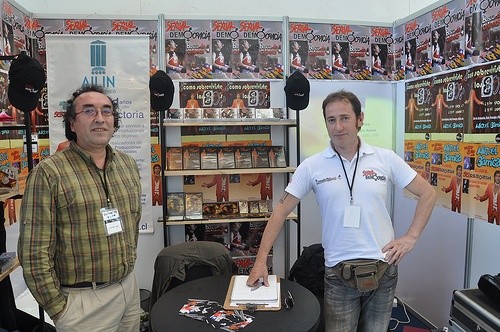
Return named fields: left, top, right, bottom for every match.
left=449, top=289, right=500, bottom=332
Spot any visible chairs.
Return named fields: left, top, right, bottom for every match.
left=288, top=244, right=325, bottom=297
left=151, top=241, right=238, bottom=306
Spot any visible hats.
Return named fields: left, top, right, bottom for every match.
left=8, top=54, right=45, bottom=111
left=286, top=71, right=311, bottom=110
left=150, top=70, right=174, bottom=111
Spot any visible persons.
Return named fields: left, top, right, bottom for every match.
left=247, top=93, right=436, bottom=332
left=18, top=85, right=143, bottom=332
left=0, top=24, right=500, bottom=225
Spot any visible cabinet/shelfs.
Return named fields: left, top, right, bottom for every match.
left=0, top=51, right=44, bottom=324
left=160, top=74, right=300, bottom=280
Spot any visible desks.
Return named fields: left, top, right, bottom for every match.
left=151, top=275, right=322, bottom=332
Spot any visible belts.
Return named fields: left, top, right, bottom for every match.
left=60, top=282, right=105, bottom=287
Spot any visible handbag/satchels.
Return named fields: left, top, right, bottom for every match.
left=331, top=259, right=390, bottom=292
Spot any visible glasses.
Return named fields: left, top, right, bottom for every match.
left=73, top=107, right=116, bottom=120
